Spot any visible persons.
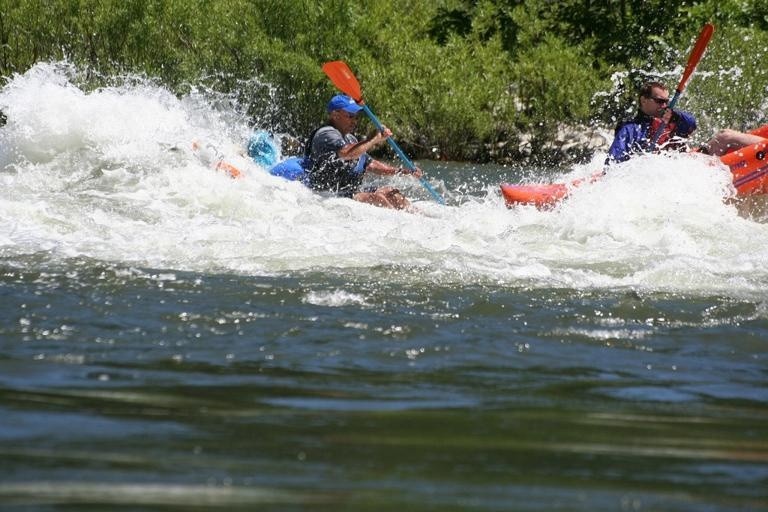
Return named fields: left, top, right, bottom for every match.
left=301, top=92, right=424, bottom=217
left=601, top=83, right=768, bottom=179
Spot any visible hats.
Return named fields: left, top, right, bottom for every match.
left=326, top=95, right=364, bottom=113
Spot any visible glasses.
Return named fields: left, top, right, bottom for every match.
left=647, top=96, right=669, bottom=104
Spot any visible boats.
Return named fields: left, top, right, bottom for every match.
left=193, top=130, right=304, bottom=182
left=500, top=123, right=768, bottom=211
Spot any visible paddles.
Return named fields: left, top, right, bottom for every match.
left=323, top=60, right=446, bottom=203
left=649, top=24, right=714, bottom=152
